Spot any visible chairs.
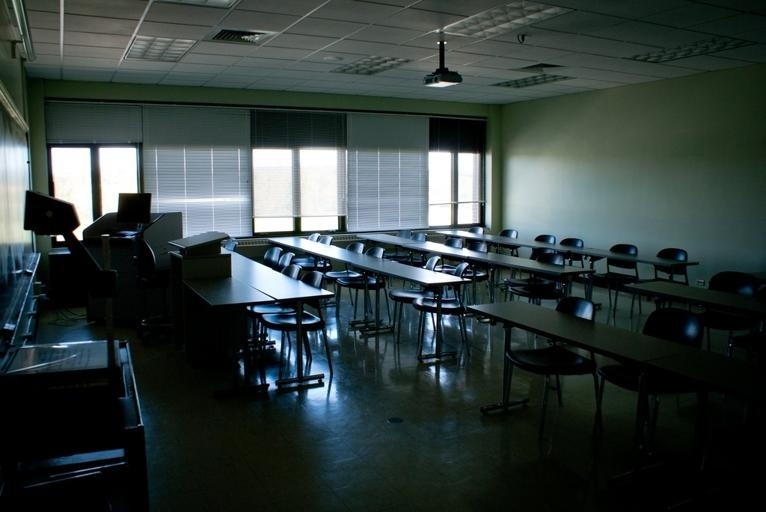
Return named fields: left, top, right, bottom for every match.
left=389, top=255, right=441, bottom=340
left=297, top=235, right=333, bottom=272
left=587, top=244, right=642, bottom=317
left=335, top=247, right=391, bottom=324
left=504, top=295, right=608, bottom=458
left=322, top=243, right=365, bottom=306
left=263, top=247, right=282, bottom=269
left=413, top=261, right=468, bottom=339
left=384, top=229, right=409, bottom=259
left=507, top=253, right=569, bottom=307
left=465, top=226, right=482, bottom=244
left=275, top=252, right=295, bottom=274
left=560, top=238, right=585, bottom=267
left=701, top=271, right=764, bottom=355
left=593, top=306, right=707, bottom=445
left=246, top=263, right=302, bottom=315
left=405, top=232, right=428, bottom=267
left=241, top=271, right=328, bottom=340
left=454, top=241, right=496, bottom=305
left=496, top=229, right=519, bottom=257
left=292, top=233, right=319, bottom=264
left=529, top=235, right=557, bottom=264
left=642, top=248, right=692, bottom=318
left=503, top=248, right=554, bottom=301
left=422, top=238, right=462, bottom=276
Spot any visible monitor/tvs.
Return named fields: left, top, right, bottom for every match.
left=116, top=193, right=151, bottom=225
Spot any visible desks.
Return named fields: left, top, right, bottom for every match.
left=442, top=230, right=701, bottom=315
left=83, top=212, right=184, bottom=314
left=268, top=237, right=473, bottom=365
left=172, top=240, right=336, bottom=384
left=1, top=340, right=150, bottom=510
left=358, top=232, right=596, bottom=341
left=620, top=280, right=761, bottom=340
left=462, top=300, right=687, bottom=447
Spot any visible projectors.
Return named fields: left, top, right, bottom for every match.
left=423, top=70, right=462, bottom=88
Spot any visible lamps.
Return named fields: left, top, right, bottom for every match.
left=423, top=3, right=462, bottom=89
